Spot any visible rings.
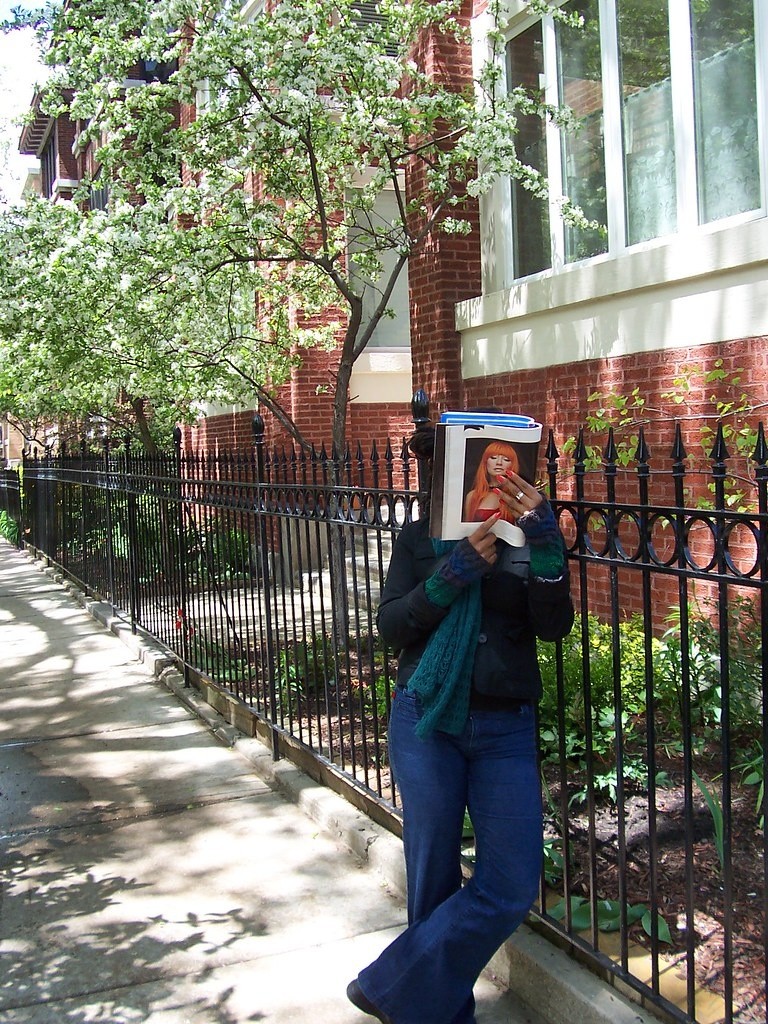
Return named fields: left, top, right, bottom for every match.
left=515, top=491, right=525, bottom=500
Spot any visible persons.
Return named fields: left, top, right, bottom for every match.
left=347, top=405, right=574, bottom=1024
left=463, top=440, right=520, bottom=526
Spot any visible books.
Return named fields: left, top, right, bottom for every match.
left=428, top=410, right=543, bottom=549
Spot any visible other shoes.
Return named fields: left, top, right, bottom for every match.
left=346, top=979, right=393, bottom=1024
